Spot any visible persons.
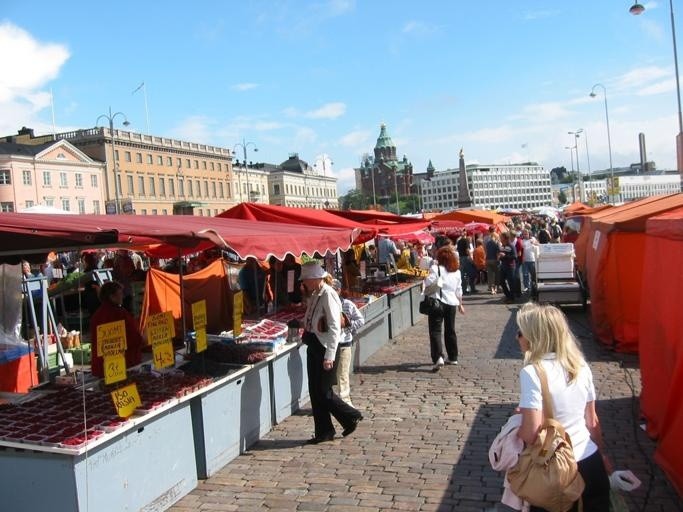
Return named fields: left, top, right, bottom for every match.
left=298, top=262, right=363, bottom=444
left=92, top=281, right=142, bottom=376
left=425, top=246, right=465, bottom=373
left=327, top=279, right=365, bottom=409
left=21, top=214, right=562, bottom=316
left=513, top=302, right=613, bottom=512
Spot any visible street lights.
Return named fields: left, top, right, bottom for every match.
left=313, top=154, right=333, bottom=209
left=589, top=83, right=615, bottom=206
left=563, top=127, right=593, bottom=205
left=231, top=137, right=258, bottom=202
left=628, top=1, right=683, bottom=196
left=390, top=166, right=431, bottom=215
left=359, top=144, right=385, bottom=211
left=92, top=106, right=130, bottom=212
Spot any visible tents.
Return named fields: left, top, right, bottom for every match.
left=564, top=194, right=683, bottom=498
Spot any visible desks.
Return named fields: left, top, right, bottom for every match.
left=0, top=271, right=424, bottom=511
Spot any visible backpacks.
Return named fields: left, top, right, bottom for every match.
left=506, top=416, right=586, bottom=511
left=419, top=298, right=446, bottom=318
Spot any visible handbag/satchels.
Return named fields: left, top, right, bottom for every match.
left=318, top=312, right=352, bottom=332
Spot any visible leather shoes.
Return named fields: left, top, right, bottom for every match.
left=307, top=432, right=336, bottom=444
left=341, top=415, right=364, bottom=437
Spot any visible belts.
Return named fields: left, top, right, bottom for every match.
left=339, top=340, right=354, bottom=347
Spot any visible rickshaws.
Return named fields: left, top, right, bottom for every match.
left=529, top=267, right=588, bottom=310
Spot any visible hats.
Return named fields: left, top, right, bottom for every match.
left=297, top=261, right=329, bottom=281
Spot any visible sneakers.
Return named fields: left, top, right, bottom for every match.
left=433, top=356, right=444, bottom=371
left=463, top=285, right=538, bottom=305
left=446, top=357, right=458, bottom=365
